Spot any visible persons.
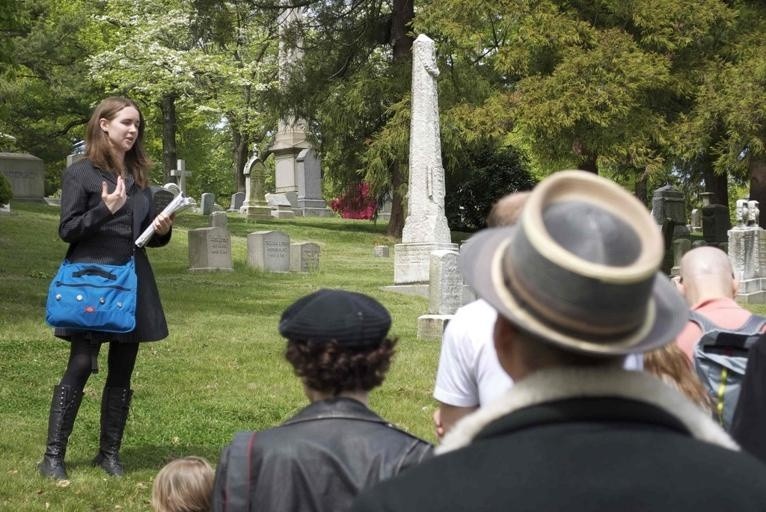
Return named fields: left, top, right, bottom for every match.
left=673, top=244, right=765, bottom=365
left=36, top=96, right=175, bottom=481
left=644, top=344, right=719, bottom=421
left=150, top=455, right=213, bottom=511
left=434, top=189, right=542, bottom=438
left=352, top=168, right=764, bottom=509
left=213, top=288, right=434, bottom=511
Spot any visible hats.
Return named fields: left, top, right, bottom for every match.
left=455, top=168, right=690, bottom=355
left=277, top=287, right=393, bottom=351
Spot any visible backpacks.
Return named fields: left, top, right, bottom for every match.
left=687, top=308, right=765, bottom=431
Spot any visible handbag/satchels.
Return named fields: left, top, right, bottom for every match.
left=47, top=263, right=138, bottom=334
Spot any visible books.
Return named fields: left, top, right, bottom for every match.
left=134, top=192, right=198, bottom=249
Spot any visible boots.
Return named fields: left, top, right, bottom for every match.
left=38, top=384, right=84, bottom=480
left=92, top=387, right=133, bottom=478
